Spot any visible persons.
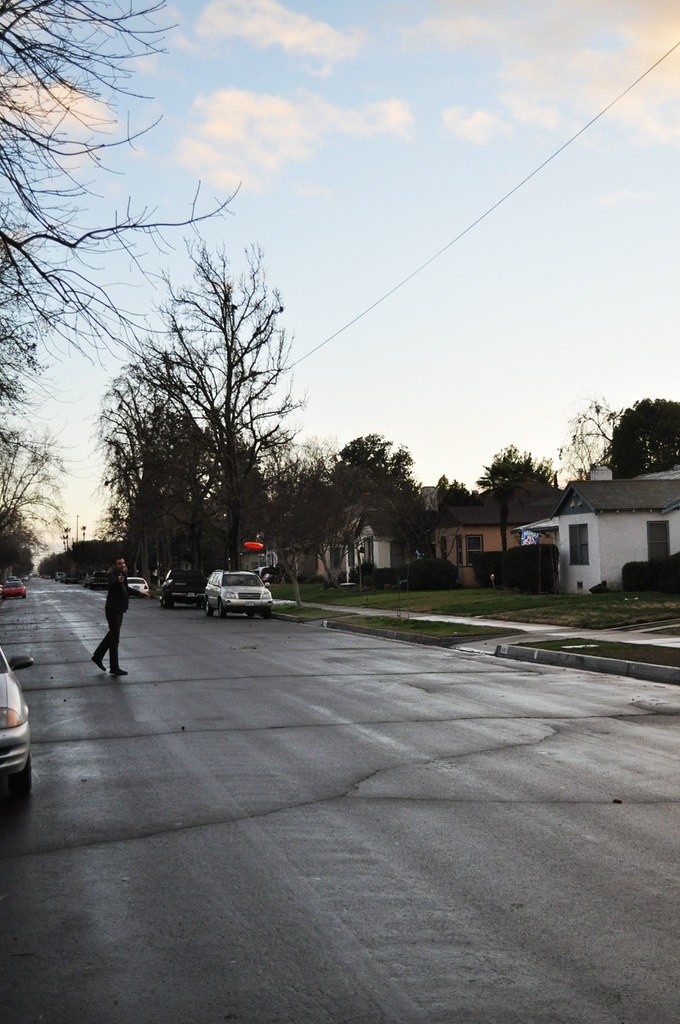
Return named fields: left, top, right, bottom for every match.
left=90, top=554, right=149, bottom=677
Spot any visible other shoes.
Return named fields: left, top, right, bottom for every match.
left=109, top=669, right=128, bottom=675
left=91, top=656, right=106, bottom=671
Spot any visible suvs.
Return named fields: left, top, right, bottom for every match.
left=91, top=571, right=110, bottom=590
left=205, top=569, right=273, bottom=619
left=84, top=572, right=93, bottom=587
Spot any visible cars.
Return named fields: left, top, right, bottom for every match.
left=0, top=580, right=28, bottom=600
left=0, top=645, right=35, bottom=796
left=6, top=568, right=80, bottom=584
left=125, top=577, right=150, bottom=598
left=254, top=566, right=273, bottom=582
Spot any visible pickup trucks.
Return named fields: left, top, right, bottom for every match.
left=161, top=569, right=210, bottom=609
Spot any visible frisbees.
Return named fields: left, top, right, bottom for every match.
left=244, top=542, right=263, bottom=549
